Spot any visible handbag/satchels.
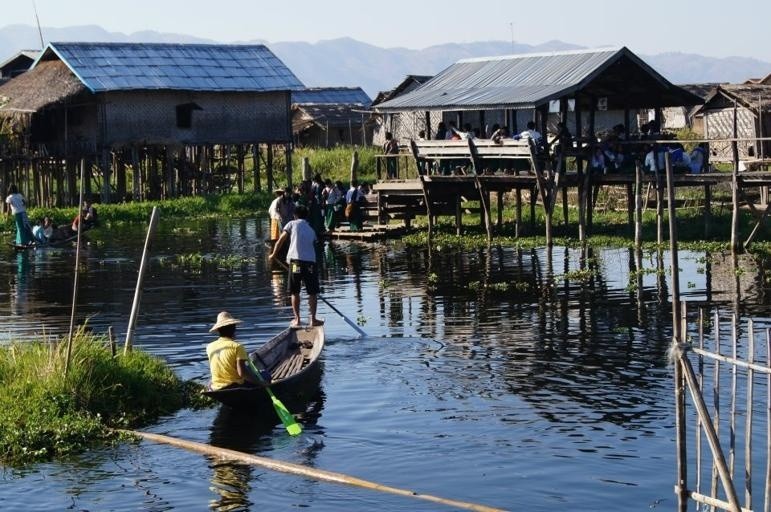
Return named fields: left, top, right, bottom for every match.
left=345, top=203, right=352, bottom=218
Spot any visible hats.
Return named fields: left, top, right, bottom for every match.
left=209, top=311, right=245, bottom=333
left=273, top=188, right=285, bottom=193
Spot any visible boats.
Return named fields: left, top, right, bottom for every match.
left=5, top=220, right=99, bottom=252
left=197, top=316, right=327, bottom=418
left=202, top=364, right=325, bottom=467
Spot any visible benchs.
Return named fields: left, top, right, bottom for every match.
left=404, top=138, right=602, bottom=160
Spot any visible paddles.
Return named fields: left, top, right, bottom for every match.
left=274, top=257, right=367, bottom=337
left=247, top=357, right=301, bottom=436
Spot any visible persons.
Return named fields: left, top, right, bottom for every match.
left=382, top=132, right=399, bottom=178
left=33, top=199, right=97, bottom=243
left=416, top=121, right=710, bottom=179
left=268, top=174, right=376, bottom=239
left=5, top=185, right=34, bottom=244
left=269, top=205, right=324, bottom=326
left=206, top=312, right=271, bottom=391
